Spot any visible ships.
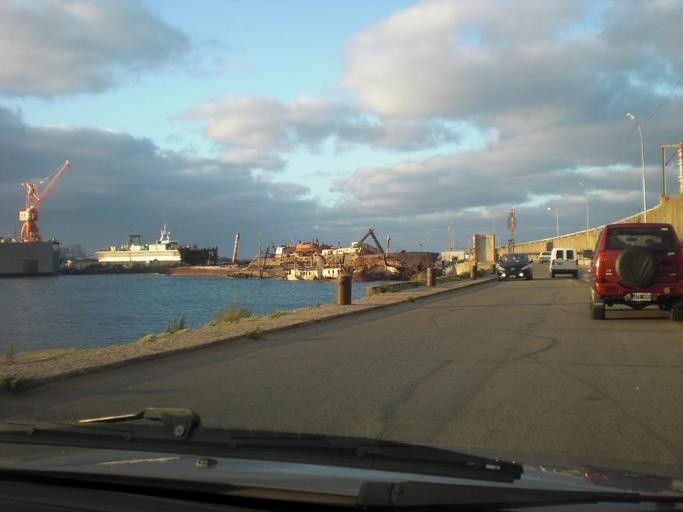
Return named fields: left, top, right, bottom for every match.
left=94, top=223, right=182, bottom=268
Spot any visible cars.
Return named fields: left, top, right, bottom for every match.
left=495, top=253, right=532, bottom=282
left=538, top=251, right=551, bottom=264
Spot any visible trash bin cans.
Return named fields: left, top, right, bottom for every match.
left=491, top=263, right=496, bottom=274
left=470, top=266, right=476, bottom=279
left=336, top=276, right=351, bottom=305
left=427, top=268, right=435, bottom=286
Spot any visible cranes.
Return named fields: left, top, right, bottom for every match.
left=19, top=160, right=69, bottom=243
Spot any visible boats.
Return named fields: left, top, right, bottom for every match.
left=178, top=244, right=217, bottom=266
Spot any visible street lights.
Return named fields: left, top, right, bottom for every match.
left=547, top=207, right=560, bottom=247
left=624, top=112, right=647, bottom=224
left=578, top=182, right=588, bottom=250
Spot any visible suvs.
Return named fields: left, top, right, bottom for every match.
left=549, top=247, right=578, bottom=277
left=582, top=221, right=682, bottom=322
